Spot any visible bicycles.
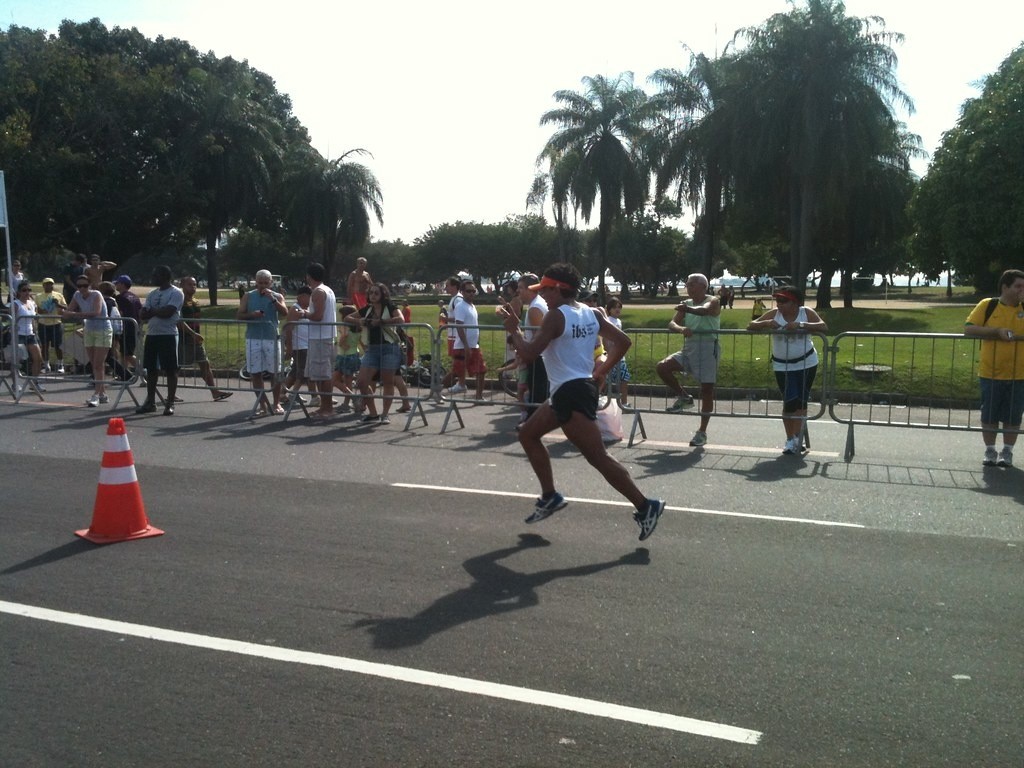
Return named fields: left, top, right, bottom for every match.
left=400, top=352, right=447, bottom=386
left=499, top=359, right=521, bottom=398
left=238, top=352, right=295, bottom=382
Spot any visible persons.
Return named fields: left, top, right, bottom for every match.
left=437, top=275, right=488, bottom=405
left=502, top=262, right=666, bottom=540
left=717, top=284, right=736, bottom=309
left=0, top=254, right=142, bottom=404
left=172, top=276, right=234, bottom=403
left=495, top=273, right=634, bottom=431
left=752, top=298, right=766, bottom=319
left=656, top=273, right=721, bottom=446
left=236, top=258, right=411, bottom=426
left=964, top=270, right=1024, bottom=468
left=747, top=286, right=828, bottom=454
left=135, top=263, right=185, bottom=416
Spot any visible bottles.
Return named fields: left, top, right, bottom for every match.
left=440, top=311, right=445, bottom=326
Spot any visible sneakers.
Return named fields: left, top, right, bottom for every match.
left=690, top=431, right=708, bottom=446
left=998, top=450, right=1012, bottom=465
left=782, top=437, right=800, bottom=454
left=983, top=448, right=999, bottom=466
left=667, top=394, right=695, bottom=413
left=632, top=497, right=666, bottom=541
left=525, top=492, right=568, bottom=523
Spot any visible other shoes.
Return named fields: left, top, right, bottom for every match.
left=622, top=403, right=635, bottom=414
left=14, top=365, right=532, bottom=424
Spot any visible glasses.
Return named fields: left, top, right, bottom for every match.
left=776, top=298, right=789, bottom=303
left=369, top=290, right=380, bottom=296
left=19, top=288, right=31, bottom=292
left=78, top=284, right=90, bottom=289
left=463, top=289, right=476, bottom=293
left=13, top=264, right=20, bottom=267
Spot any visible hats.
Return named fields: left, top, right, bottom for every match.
left=42, top=278, right=54, bottom=285
left=527, top=275, right=577, bottom=290
left=111, top=275, right=132, bottom=285
left=772, top=291, right=801, bottom=305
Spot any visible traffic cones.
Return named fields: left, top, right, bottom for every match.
left=70, top=418, right=170, bottom=546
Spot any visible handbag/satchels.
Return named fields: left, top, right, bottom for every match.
left=595, top=395, right=622, bottom=443
left=397, top=328, right=414, bottom=353
left=106, top=298, right=123, bottom=334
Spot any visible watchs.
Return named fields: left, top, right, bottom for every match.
left=799, top=322, right=805, bottom=328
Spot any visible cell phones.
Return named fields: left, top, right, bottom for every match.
left=1007, top=331, right=1013, bottom=339
